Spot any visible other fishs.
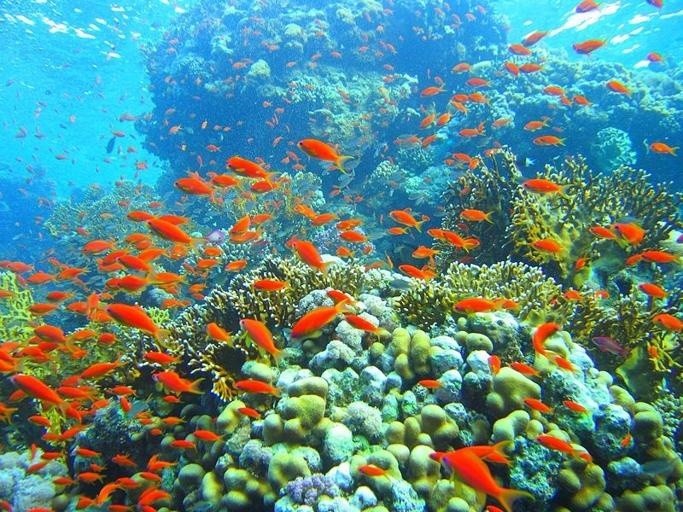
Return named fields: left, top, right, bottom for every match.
left=0, top=0, right=682, bottom=512
